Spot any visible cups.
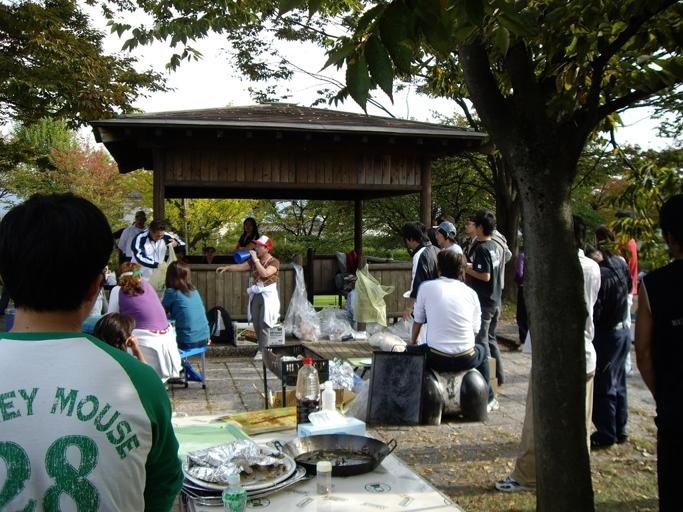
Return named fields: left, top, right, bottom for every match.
left=466, top=263, right=472, bottom=270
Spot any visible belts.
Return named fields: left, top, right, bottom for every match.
left=613, top=319, right=629, bottom=332
left=429, top=348, right=475, bottom=358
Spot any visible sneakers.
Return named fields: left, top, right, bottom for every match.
left=253, top=349, right=264, bottom=361
left=494, top=475, right=537, bottom=494
left=486, top=398, right=499, bottom=413
left=590, top=432, right=630, bottom=452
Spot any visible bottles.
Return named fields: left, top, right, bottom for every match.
left=221, top=473, right=247, bottom=512
left=7, top=298, right=15, bottom=314
left=295, top=358, right=320, bottom=433
left=321, top=381, right=336, bottom=410
left=315, top=461, right=333, bottom=494
left=629, top=294, right=638, bottom=341
left=396, top=309, right=413, bottom=333
left=103, top=266, right=115, bottom=285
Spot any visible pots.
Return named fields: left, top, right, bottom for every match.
left=274, top=434, right=397, bottom=476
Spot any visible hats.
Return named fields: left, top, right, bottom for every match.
left=432, top=221, right=457, bottom=238
left=135, top=211, right=146, bottom=217
left=250, top=235, right=273, bottom=250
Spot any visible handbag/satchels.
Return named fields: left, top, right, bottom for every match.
left=207, top=305, right=235, bottom=345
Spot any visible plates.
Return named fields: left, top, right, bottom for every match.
left=182, top=446, right=296, bottom=490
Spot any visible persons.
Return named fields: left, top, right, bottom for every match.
left=495, top=213, right=638, bottom=491
left=216, top=218, right=280, bottom=359
left=0, top=189, right=184, bottom=511
left=403, top=211, right=513, bottom=412
left=90, top=210, right=211, bottom=383
left=633, top=193, right=682, bottom=511
